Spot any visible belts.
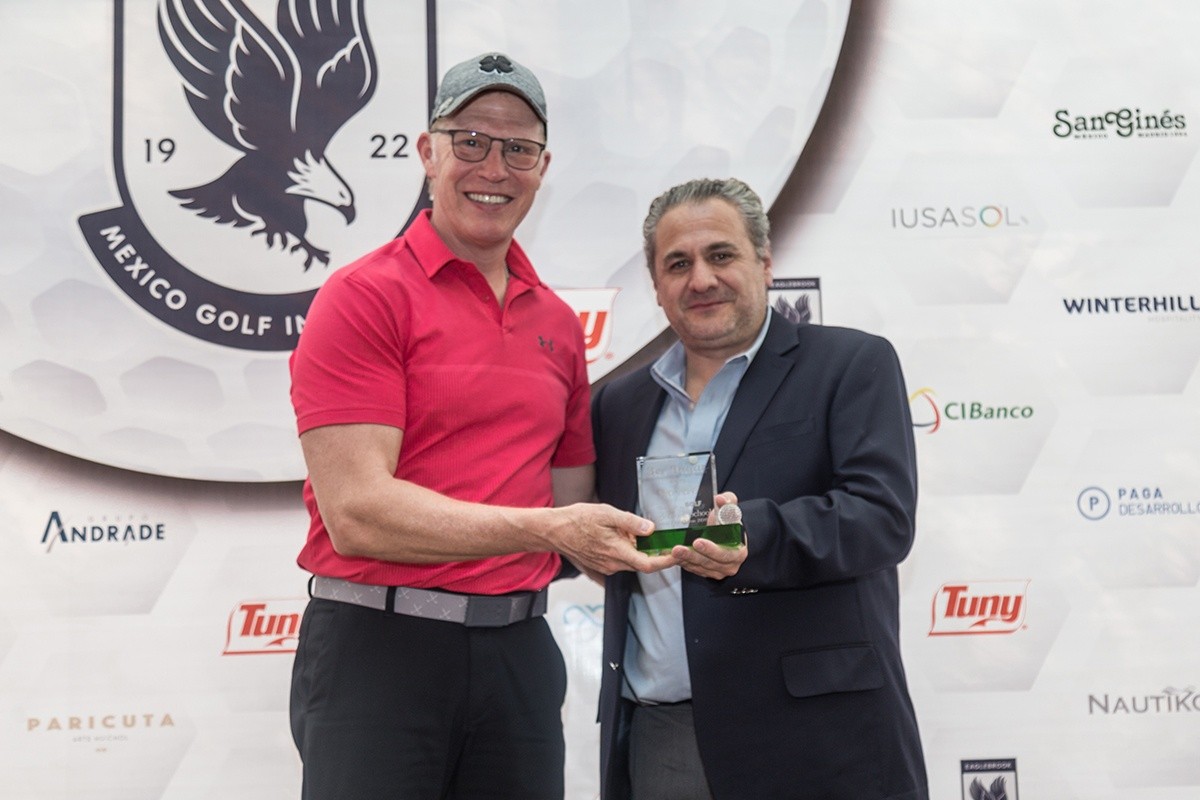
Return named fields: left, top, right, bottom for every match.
left=314, top=575, right=548, bottom=626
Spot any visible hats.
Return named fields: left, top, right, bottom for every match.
left=429, top=53, right=547, bottom=128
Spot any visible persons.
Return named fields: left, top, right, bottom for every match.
left=550, top=178, right=932, bottom=800
left=288, top=54, right=689, bottom=800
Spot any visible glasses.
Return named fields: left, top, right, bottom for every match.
left=429, top=129, right=546, bottom=170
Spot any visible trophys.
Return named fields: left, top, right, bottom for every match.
left=629, top=452, right=745, bottom=557
